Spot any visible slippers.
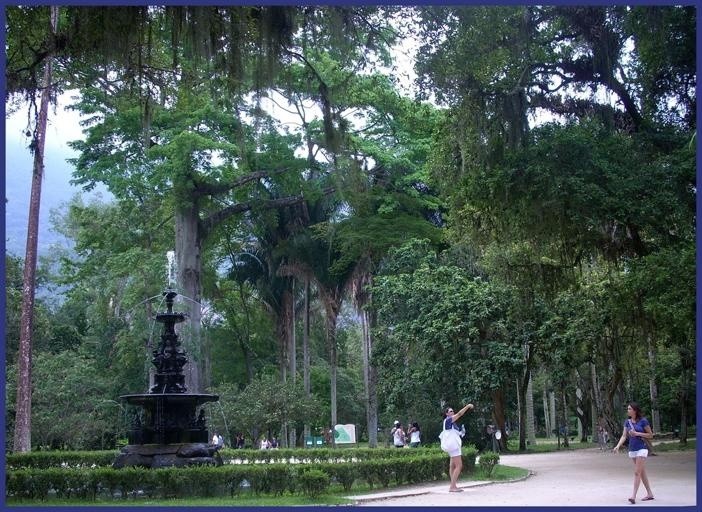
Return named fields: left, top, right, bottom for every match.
left=628, top=498, right=635, bottom=503
left=641, top=497, right=653, bottom=500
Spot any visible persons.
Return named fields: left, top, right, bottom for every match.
left=211, top=431, right=225, bottom=449
left=393, top=423, right=406, bottom=449
left=440, top=404, right=475, bottom=492
left=390, top=421, right=403, bottom=436
left=235, top=432, right=245, bottom=449
left=407, top=422, right=421, bottom=449
left=260, top=436, right=271, bottom=449
left=268, top=436, right=279, bottom=450
left=612, top=403, right=655, bottom=504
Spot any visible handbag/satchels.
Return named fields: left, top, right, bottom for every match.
left=438, top=418, right=461, bottom=452
left=640, top=437, right=656, bottom=456
